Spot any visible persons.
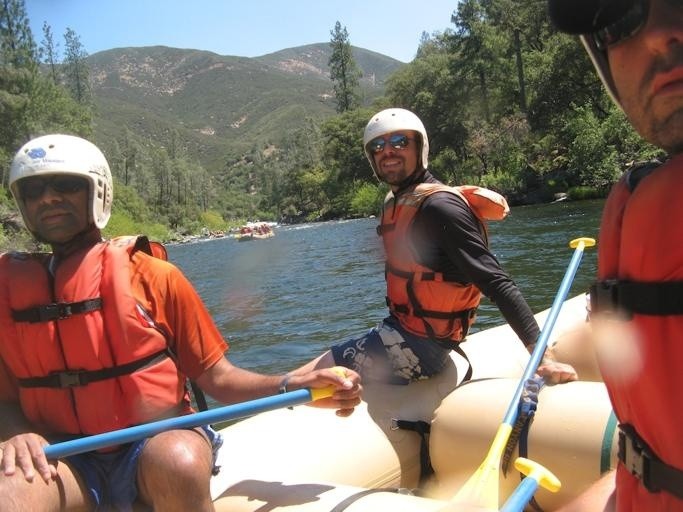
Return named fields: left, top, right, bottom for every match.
left=1, top=132, right=363, bottom=512
left=531, top=0, right=683, bottom=512
left=241, top=224, right=270, bottom=237
left=286, top=106, right=578, bottom=384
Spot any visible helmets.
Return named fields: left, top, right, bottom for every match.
left=8, top=134, right=114, bottom=232
left=547, top=1, right=625, bottom=115
left=363, top=107, right=430, bottom=183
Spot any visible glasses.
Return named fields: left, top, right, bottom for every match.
left=20, top=173, right=88, bottom=199
left=585, top=2, right=650, bottom=49
left=367, top=135, right=412, bottom=154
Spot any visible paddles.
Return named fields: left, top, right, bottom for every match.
left=447, top=238, right=597, bottom=511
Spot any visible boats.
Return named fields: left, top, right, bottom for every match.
left=233, top=229, right=274, bottom=241
left=206, top=285, right=605, bottom=512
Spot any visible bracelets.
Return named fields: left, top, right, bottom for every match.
left=278, top=374, right=294, bottom=411
